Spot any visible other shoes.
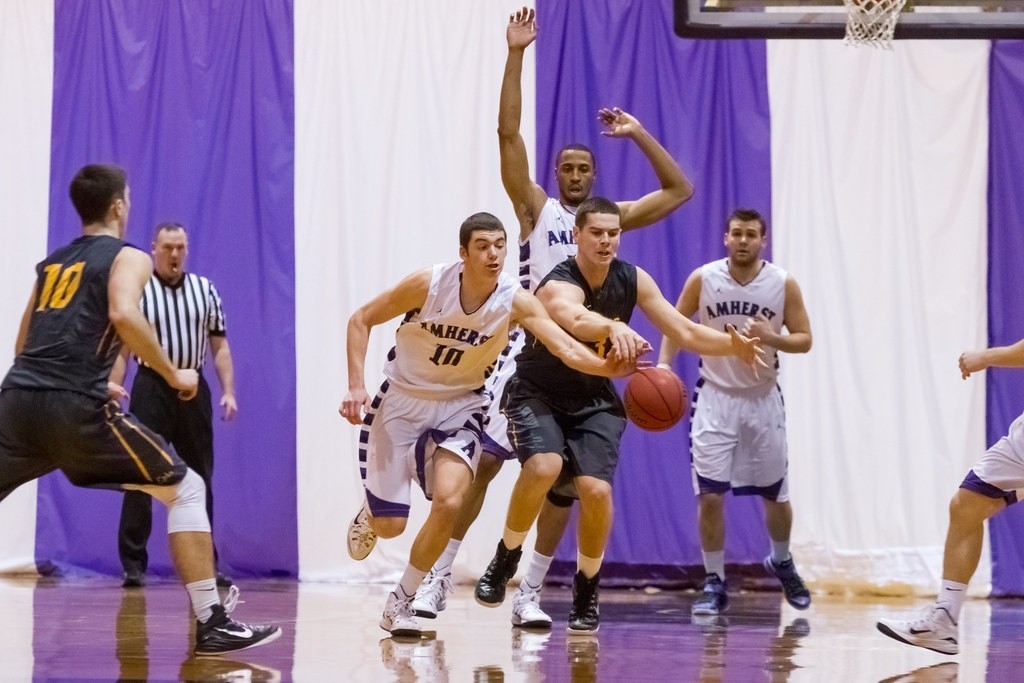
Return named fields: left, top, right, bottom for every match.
left=121, top=570, right=145, bottom=587
left=215, top=570, right=233, bottom=585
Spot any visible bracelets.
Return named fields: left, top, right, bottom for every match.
left=658, top=363, right=672, bottom=372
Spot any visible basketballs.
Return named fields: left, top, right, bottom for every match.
left=624, top=368, right=688, bottom=431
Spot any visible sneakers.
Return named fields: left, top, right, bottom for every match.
left=379, top=591, right=422, bottom=637
left=412, top=566, right=455, bottom=619
left=511, top=586, right=553, bottom=628
left=474, top=538, right=523, bottom=609
left=763, top=550, right=810, bottom=610
left=691, top=571, right=731, bottom=615
left=876, top=596, right=959, bottom=655
left=193, top=584, right=283, bottom=654
left=346, top=496, right=378, bottom=560
left=565, top=570, right=601, bottom=636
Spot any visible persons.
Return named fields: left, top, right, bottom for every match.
left=108, top=223, right=240, bottom=588
left=497, top=7, right=695, bottom=627
left=876, top=337, right=1024, bottom=655
left=658, top=208, right=810, bottom=613
left=474, top=199, right=771, bottom=635
left=340, top=213, right=654, bottom=637
left=0, top=165, right=283, bottom=656
